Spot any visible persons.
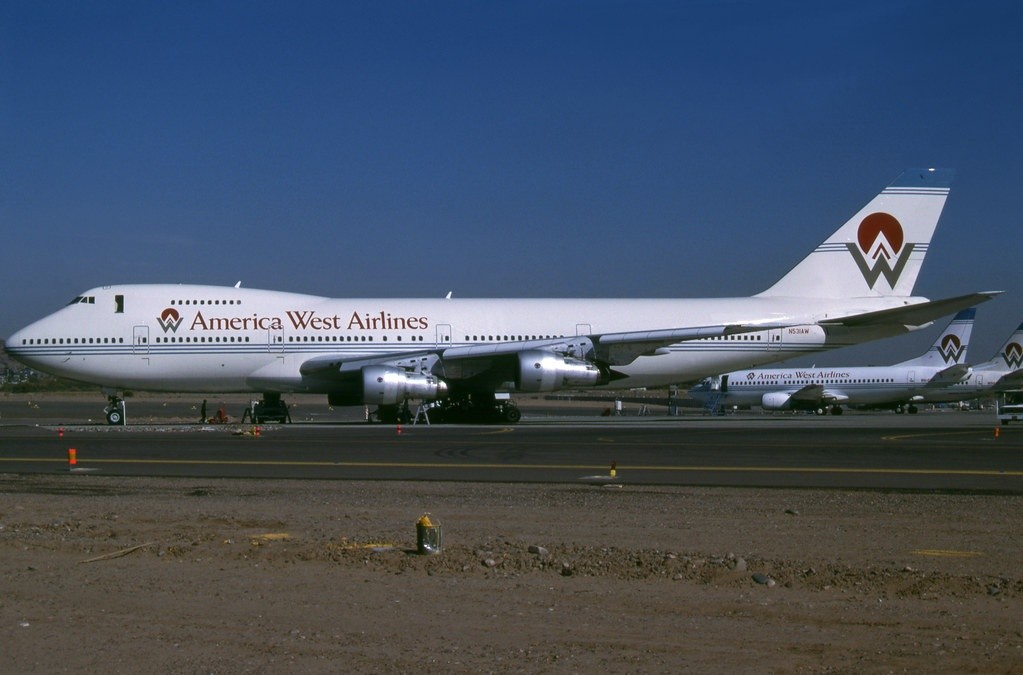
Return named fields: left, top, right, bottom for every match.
left=199, top=398, right=208, bottom=425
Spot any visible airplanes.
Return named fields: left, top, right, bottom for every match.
left=7, top=186, right=1006, bottom=423
left=689, top=308, right=1023, bottom=415
